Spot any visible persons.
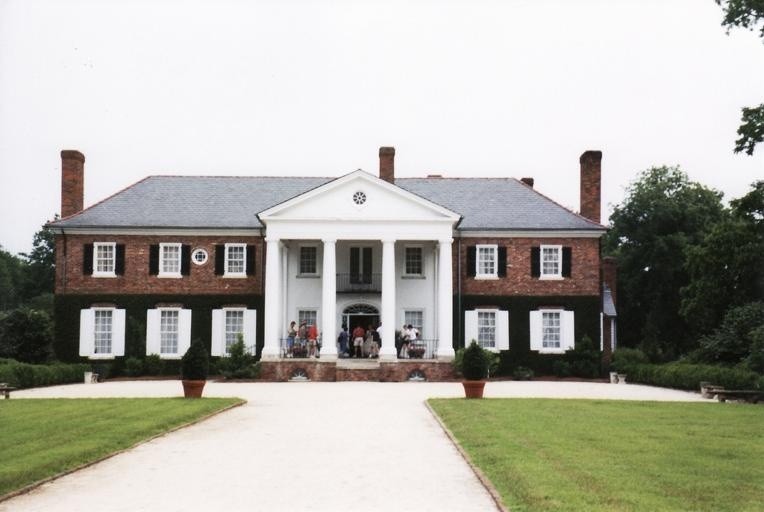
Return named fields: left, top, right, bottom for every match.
left=285, top=319, right=421, bottom=359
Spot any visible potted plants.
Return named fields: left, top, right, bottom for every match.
left=177, top=336, right=211, bottom=399
left=82, top=362, right=99, bottom=384
left=459, top=339, right=491, bottom=398
left=609, top=359, right=631, bottom=385
left=699, top=366, right=728, bottom=401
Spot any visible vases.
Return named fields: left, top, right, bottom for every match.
left=410, top=356, right=421, bottom=359
left=293, top=354, right=304, bottom=358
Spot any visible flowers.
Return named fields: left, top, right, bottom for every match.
left=406, top=348, right=425, bottom=358
left=293, top=347, right=308, bottom=355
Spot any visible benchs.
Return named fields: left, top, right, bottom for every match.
left=707, top=389, right=764, bottom=404
left=0, top=386, right=17, bottom=399
